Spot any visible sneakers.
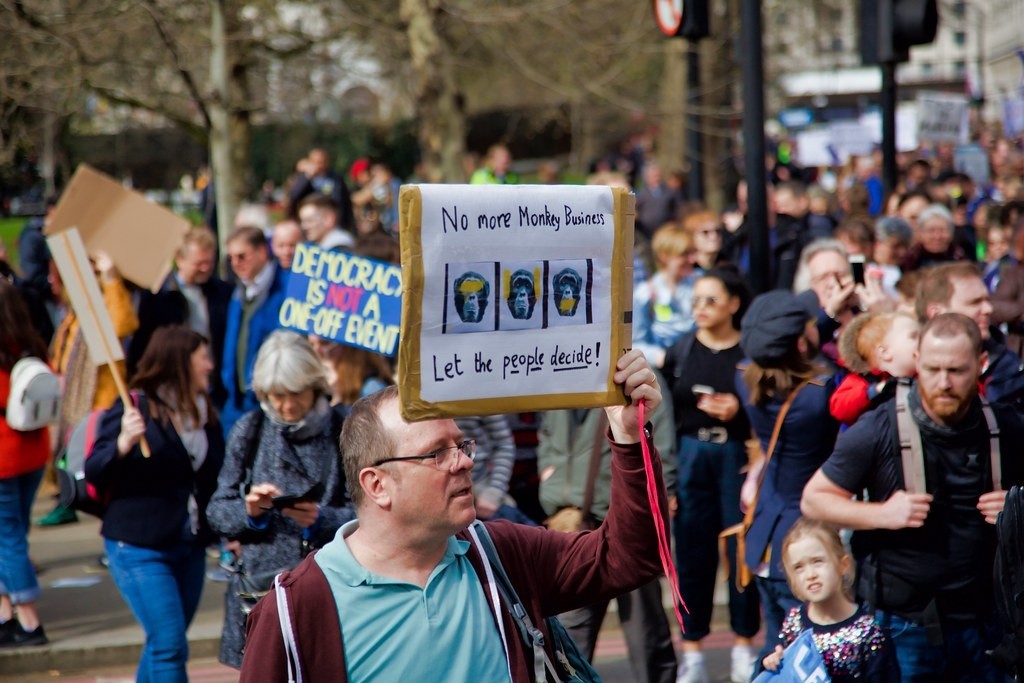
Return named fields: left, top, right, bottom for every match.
left=730, top=653, right=759, bottom=683
left=0, top=620, right=46, bottom=647
left=36, top=505, right=78, bottom=527
left=677, top=662, right=708, bottom=683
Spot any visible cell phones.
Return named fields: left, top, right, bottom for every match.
left=272, top=483, right=324, bottom=512
left=849, top=254, right=867, bottom=289
left=693, top=386, right=712, bottom=398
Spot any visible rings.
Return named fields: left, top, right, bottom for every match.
left=652, top=373, right=656, bottom=382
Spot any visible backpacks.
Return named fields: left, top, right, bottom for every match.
left=55, top=389, right=150, bottom=518
left=474, top=519, right=603, bottom=683
left=0, top=355, right=61, bottom=432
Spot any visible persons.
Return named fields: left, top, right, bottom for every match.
left=0, top=118, right=1024, bottom=683
left=235, top=347, right=673, bottom=683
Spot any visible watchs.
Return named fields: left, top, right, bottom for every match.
left=642, top=428, right=651, bottom=438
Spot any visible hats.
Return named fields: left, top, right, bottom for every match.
left=741, top=289, right=820, bottom=368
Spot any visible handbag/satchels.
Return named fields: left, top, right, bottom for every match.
left=719, top=524, right=757, bottom=588
left=238, top=565, right=295, bottom=636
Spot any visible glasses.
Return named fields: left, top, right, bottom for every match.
left=371, top=439, right=477, bottom=470
left=225, top=253, right=245, bottom=261
left=692, top=296, right=732, bottom=306
left=700, top=228, right=723, bottom=235
